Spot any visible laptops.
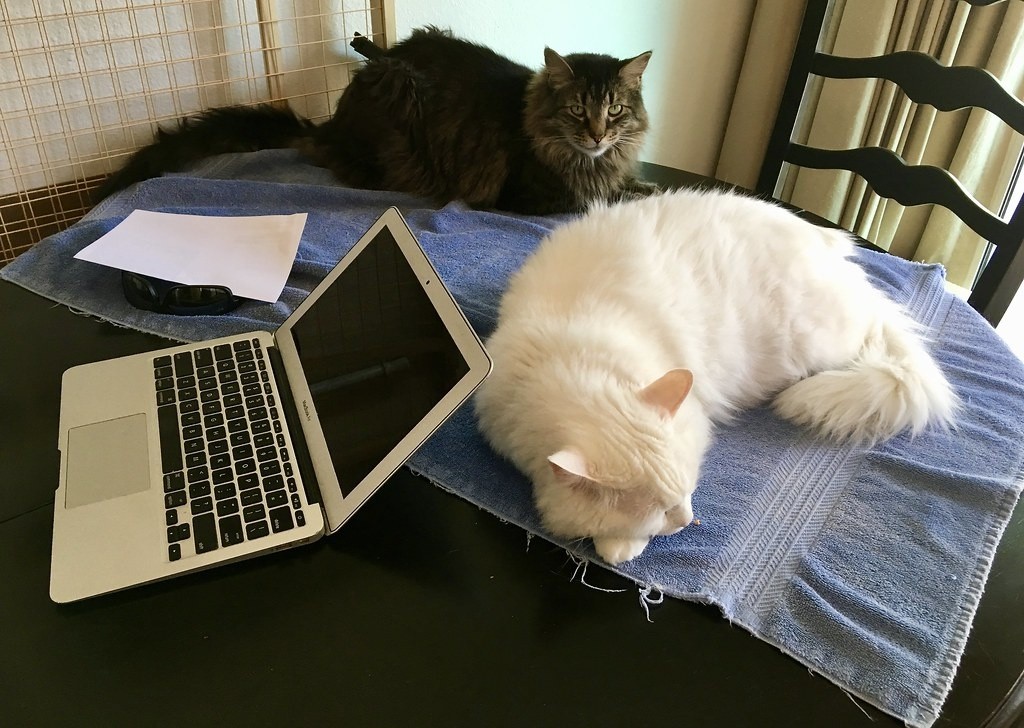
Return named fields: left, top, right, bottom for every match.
left=48, top=207, right=493, bottom=602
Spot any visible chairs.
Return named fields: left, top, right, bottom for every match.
left=754, top=0, right=1024, bottom=329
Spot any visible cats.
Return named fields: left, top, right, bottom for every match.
left=91, top=22, right=654, bottom=219
left=472, top=189, right=962, bottom=568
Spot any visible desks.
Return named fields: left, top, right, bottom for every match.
left=0, top=159, right=1024, bottom=728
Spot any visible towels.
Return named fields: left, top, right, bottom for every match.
left=0, top=136, right=1024, bottom=728
